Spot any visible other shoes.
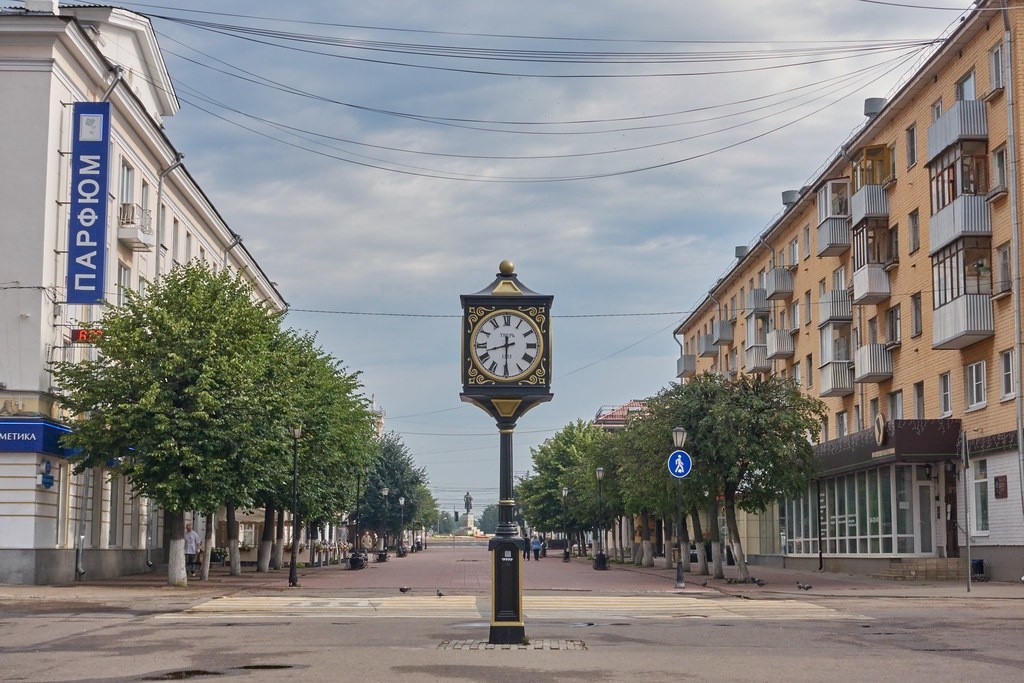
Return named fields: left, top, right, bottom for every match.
left=192, top=573, right=196, bottom=577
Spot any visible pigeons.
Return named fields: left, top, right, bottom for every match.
left=399, top=587, right=446, bottom=598
left=700, top=576, right=813, bottom=591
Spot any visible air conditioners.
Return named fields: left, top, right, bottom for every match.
left=122, top=203, right=142, bottom=225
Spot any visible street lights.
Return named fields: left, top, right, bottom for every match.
left=671, top=417, right=689, bottom=589
left=562, top=486, right=569, bottom=561
left=288, top=420, right=302, bottom=587
left=595, top=465, right=608, bottom=569
left=351, top=462, right=371, bottom=557
left=398, top=496, right=406, bottom=545
left=381, top=486, right=389, bottom=561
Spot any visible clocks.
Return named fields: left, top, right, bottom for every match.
left=458, top=259, right=553, bottom=646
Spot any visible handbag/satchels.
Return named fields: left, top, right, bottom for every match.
left=539, top=551, right=543, bottom=558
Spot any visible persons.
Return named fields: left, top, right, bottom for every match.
left=360, top=532, right=373, bottom=560
left=464, top=492, right=473, bottom=513
left=184, top=523, right=200, bottom=576
left=523, top=534, right=546, bottom=561
left=416, top=534, right=421, bottom=543
